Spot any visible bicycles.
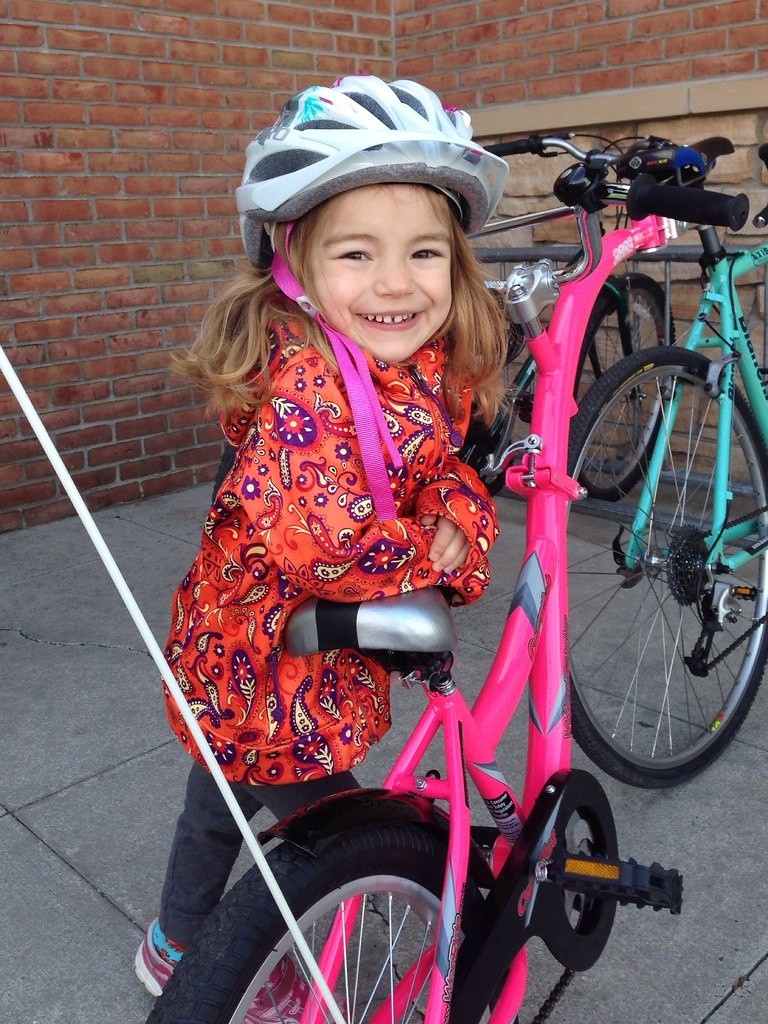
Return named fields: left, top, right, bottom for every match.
left=139, top=121, right=768, bottom=1024
left=554, top=132, right=768, bottom=792
left=457, top=120, right=679, bottom=500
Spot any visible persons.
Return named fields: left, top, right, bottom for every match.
left=134, top=74, right=510, bottom=1024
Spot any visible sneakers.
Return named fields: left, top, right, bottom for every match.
left=134, top=919, right=356, bottom=1024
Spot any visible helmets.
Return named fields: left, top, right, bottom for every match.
left=236, top=73, right=508, bottom=274
